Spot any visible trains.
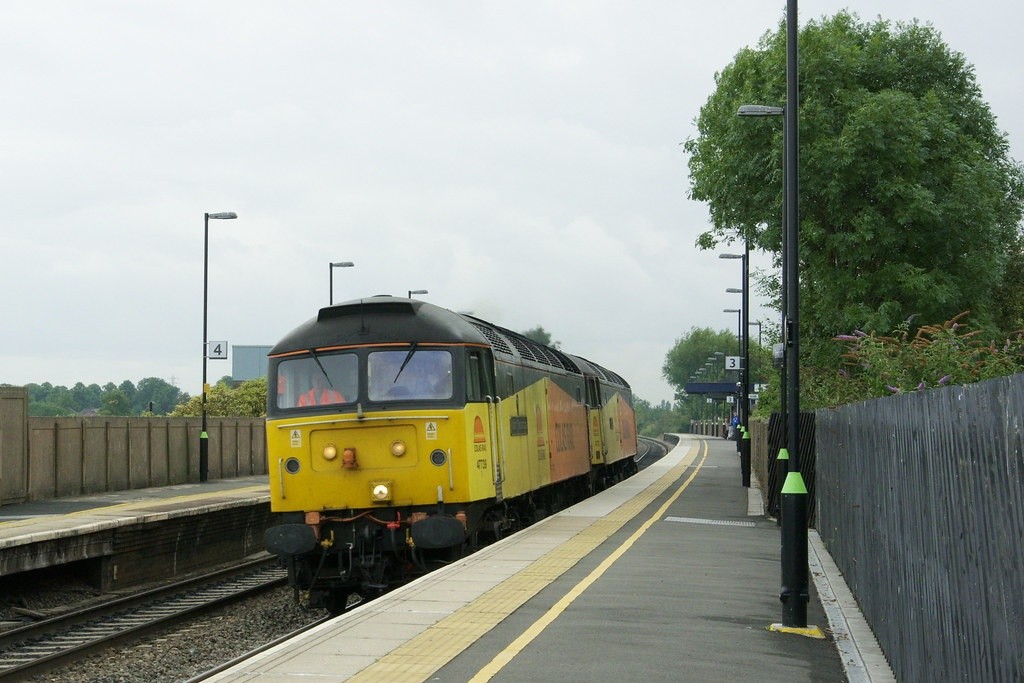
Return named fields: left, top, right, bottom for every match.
left=264, top=294, right=639, bottom=613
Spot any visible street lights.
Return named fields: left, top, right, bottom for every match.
left=330, top=262, right=354, bottom=305
left=738, top=105, right=790, bottom=527
left=674, top=254, right=762, bottom=487
left=201, top=212, right=238, bottom=482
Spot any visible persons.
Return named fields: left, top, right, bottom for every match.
left=728, top=411, right=740, bottom=438
left=296, top=372, right=346, bottom=405
